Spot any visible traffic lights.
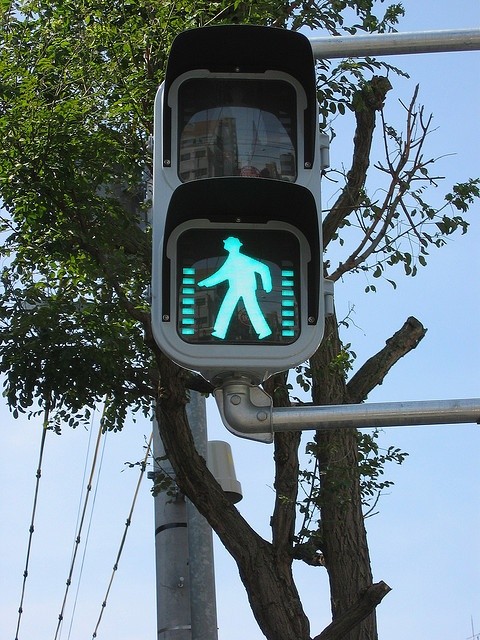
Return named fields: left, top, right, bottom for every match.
left=150, top=26, right=325, bottom=370
